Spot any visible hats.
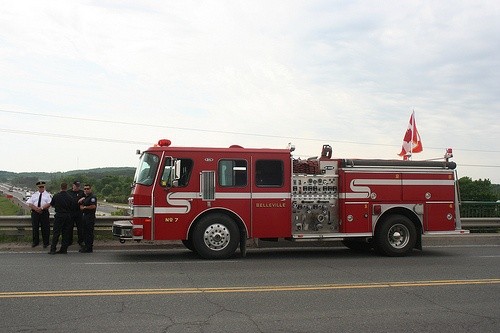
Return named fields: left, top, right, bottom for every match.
left=36, top=181, right=45, bottom=187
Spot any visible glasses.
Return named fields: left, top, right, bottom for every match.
left=84, top=188, right=89, bottom=190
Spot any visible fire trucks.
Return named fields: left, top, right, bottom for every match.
left=111, top=139, right=470, bottom=261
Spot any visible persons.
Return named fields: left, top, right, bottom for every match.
left=49, top=182, right=73, bottom=254
left=25, top=181, right=53, bottom=248
left=68, top=181, right=84, bottom=247
left=80, top=184, right=97, bottom=253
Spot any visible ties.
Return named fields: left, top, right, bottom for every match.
left=38, top=192, right=42, bottom=207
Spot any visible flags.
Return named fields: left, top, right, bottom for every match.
left=400, top=113, right=423, bottom=156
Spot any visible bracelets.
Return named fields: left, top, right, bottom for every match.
left=41, top=207, right=43, bottom=210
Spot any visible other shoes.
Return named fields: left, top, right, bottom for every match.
left=79, top=242, right=85, bottom=248
left=79, top=249, right=93, bottom=253
left=55, top=250, right=67, bottom=254
left=32, top=242, right=39, bottom=247
left=48, top=251, right=56, bottom=255
